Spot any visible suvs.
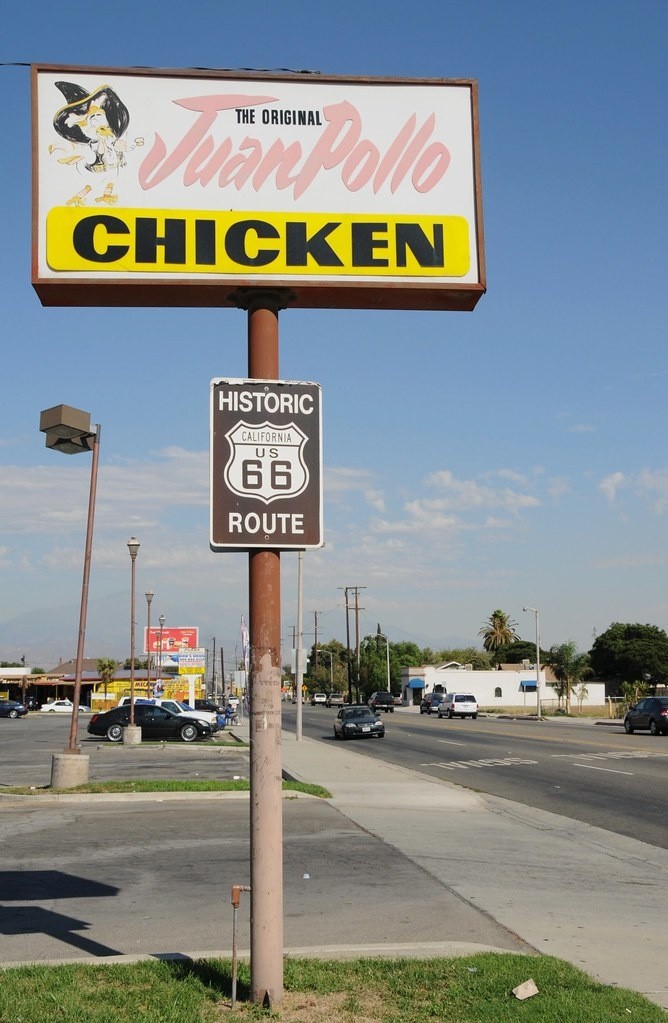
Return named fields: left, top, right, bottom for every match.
left=311, top=693, right=327, bottom=706
left=327, top=693, right=344, bottom=708
left=367, top=692, right=395, bottom=713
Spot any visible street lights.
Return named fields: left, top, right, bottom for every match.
left=127, top=537, right=142, bottom=726
left=522, top=606, right=541, bottom=716
left=155, top=631, right=161, bottom=681
left=37, top=404, right=103, bottom=756
left=317, top=650, right=334, bottom=692
left=158, top=614, right=166, bottom=699
left=144, top=590, right=155, bottom=699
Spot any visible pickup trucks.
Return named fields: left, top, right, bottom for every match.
left=153, top=699, right=218, bottom=724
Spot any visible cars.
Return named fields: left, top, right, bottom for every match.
left=437, top=693, right=479, bottom=719
left=117, top=696, right=147, bottom=708
left=87, top=703, right=212, bottom=743
left=40, top=700, right=91, bottom=713
left=420, top=693, right=446, bottom=714
left=182, top=698, right=226, bottom=714
left=394, top=693, right=403, bottom=704
left=229, top=697, right=239, bottom=705
left=333, top=706, right=385, bottom=740
left=624, top=698, right=668, bottom=736
left=292, top=696, right=305, bottom=704
left=0, top=699, right=28, bottom=719
